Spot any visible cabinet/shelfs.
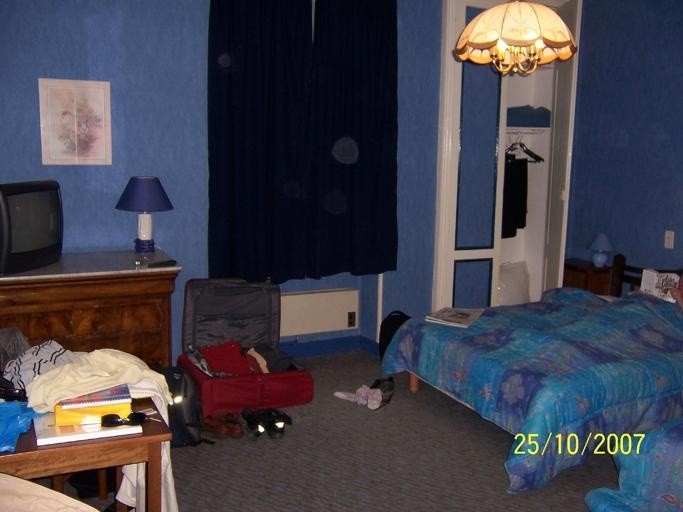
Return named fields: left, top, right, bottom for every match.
left=359, top=1, right=582, bottom=343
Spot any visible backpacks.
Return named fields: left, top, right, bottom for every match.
left=378, top=309, right=412, bottom=364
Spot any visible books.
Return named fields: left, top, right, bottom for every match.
left=423, top=307, right=486, bottom=329
left=33, top=384, right=143, bottom=448
left=640, top=267, right=679, bottom=304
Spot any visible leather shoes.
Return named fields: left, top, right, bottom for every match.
left=258, top=408, right=286, bottom=439
left=239, top=406, right=266, bottom=442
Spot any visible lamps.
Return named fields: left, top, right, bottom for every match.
left=452, top=1, right=578, bottom=75
left=115, top=176, right=175, bottom=253
left=585, top=233, right=613, bottom=267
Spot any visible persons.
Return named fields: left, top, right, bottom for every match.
left=670, top=270, right=683, bottom=305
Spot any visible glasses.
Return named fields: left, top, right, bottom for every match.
left=2, top=388, right=28, bottom=402
left=101, top=411, right=150, bottom=427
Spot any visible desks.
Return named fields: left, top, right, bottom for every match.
left=0, top=397, right=173, bottom=512
left=0, top=249, right=183, bottom=370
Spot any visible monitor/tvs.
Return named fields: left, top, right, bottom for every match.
left=0, top=181, right=64, bottom=277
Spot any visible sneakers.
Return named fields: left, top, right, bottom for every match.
left=216, top=412, right=244, bottom=438
left=198, top=415, right=230, bottom=440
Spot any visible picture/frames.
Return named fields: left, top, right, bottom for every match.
left=37, top=78, right=113, bottom=166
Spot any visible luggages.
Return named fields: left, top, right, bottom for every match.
left=177, top=273, right=316, bottom=423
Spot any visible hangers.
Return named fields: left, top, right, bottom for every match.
left=505, top=131, right=544, bottom=163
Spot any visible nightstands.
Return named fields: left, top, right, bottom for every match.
left=563, top=254, right=626, bottom=298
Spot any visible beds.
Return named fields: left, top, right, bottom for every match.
left=382, top=254, right=683, bottom=495
left=585, top=395, right=682, bottom=511
